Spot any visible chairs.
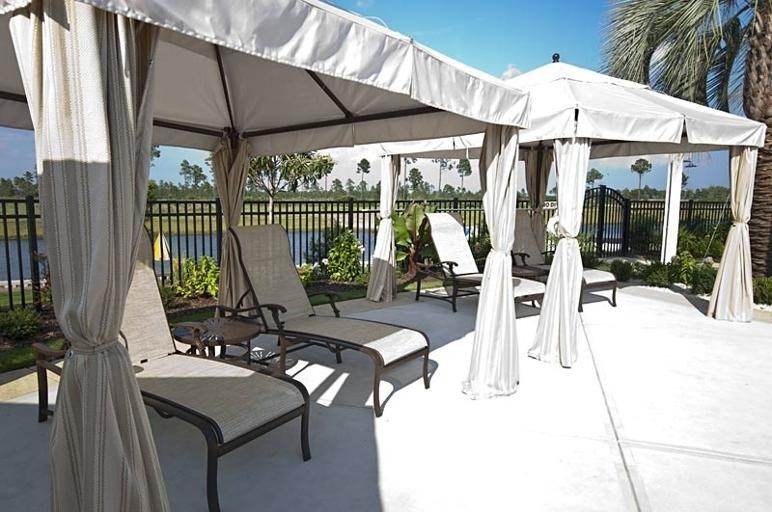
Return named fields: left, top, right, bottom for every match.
left=413, top=209, right=618, bottom=313
